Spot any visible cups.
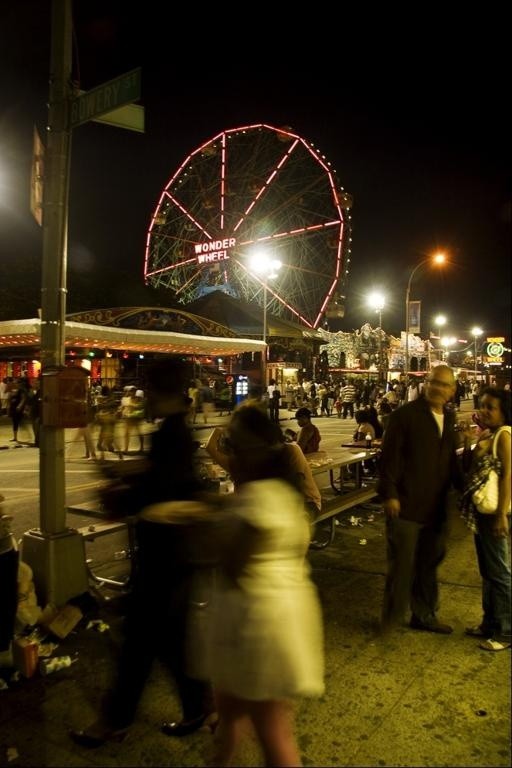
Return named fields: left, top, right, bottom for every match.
left=39, top=655, right=71, bottom=675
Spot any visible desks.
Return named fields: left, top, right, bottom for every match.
left=304, top=440, right=385, bottom=496
left=65, top=499, right=141, bottom=590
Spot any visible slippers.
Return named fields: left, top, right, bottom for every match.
left=466, top=624, right=494, bottom=636
left=480, top=638, right=512, bottom=650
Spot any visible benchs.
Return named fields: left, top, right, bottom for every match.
left=73, top=522, right=130, bottom=589
left=308, top=478, right=387, bottom=550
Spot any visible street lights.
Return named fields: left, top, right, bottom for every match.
left=433, top=314, right=446, bottom=339
left=403, top=253, right=448, bottom=371
left=376, top=293, right=385, bottom=329
left=470, top=325, right=483, bottom=371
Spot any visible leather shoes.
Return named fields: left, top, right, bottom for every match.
left=411, top=614, right=453, bottom=633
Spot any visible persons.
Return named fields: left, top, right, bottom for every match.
left=90, top=354, right=323, bottom=767
left=1, top=355, right=512, bottom=451
left=377, top=363, right=512, bottom=652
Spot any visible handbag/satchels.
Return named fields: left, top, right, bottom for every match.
left=472, top=426, right=512, bottom=515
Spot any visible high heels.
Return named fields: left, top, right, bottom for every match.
left=161, top=698, right=221, bottom=738
left=66, top=723, right=131, bottom=749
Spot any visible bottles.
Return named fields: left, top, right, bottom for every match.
left=111, top=549, right=130, bottom=560
left=218, top=468, right=226, bottom=487
left=365, top=432, right=372, bottom=451
